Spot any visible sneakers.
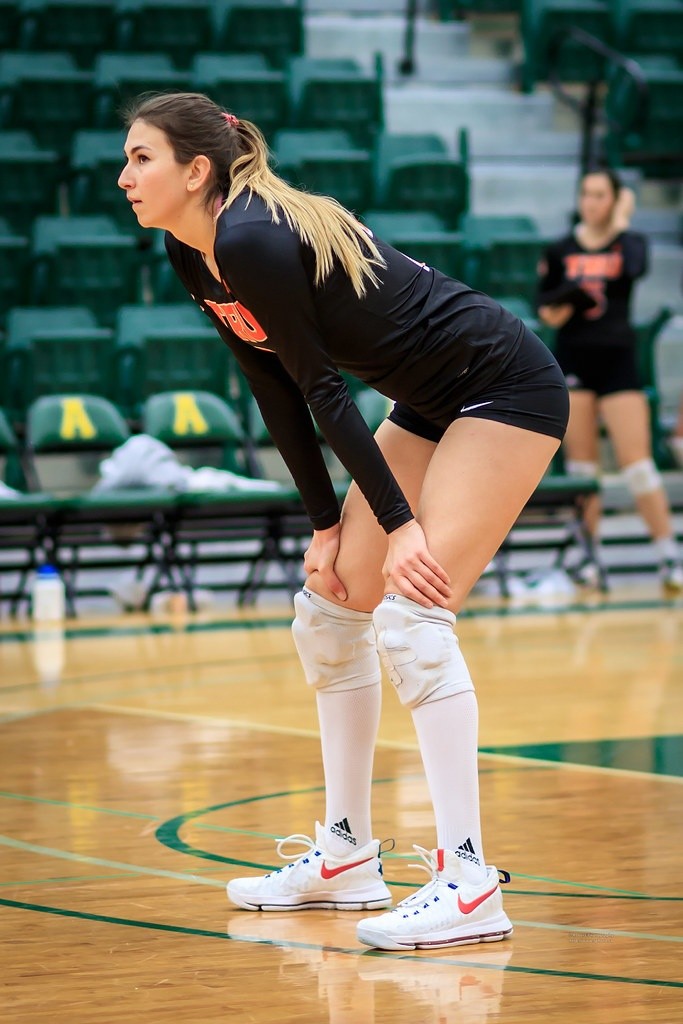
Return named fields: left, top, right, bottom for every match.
left=356, top=844, right=514, bottom=950
left=227, top=822, right=395, bottom=912
left=229, top=906, right=371, bottom=970
left=359, top=948, right=514, bottom=1001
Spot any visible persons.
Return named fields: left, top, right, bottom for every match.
left=117, top=93, right=570, bottom=950
left=536, top=168, right=683, bottom=600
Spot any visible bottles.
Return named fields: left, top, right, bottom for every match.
left=34, top=563, right=66, bottom=623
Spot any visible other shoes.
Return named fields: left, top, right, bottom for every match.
left=579, top=562, right=609, bottom=593
left=657, top=545, right=683, bottom=594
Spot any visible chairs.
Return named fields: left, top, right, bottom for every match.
left=0, top=0, right=683, bottom=621
